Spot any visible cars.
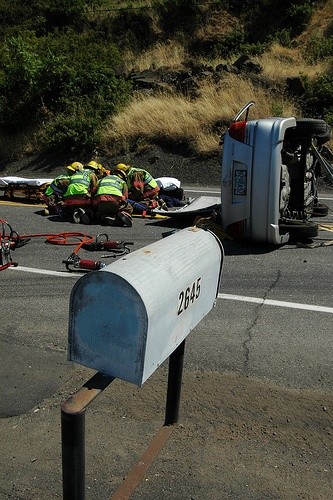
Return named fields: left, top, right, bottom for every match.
left=152, top=100, right=331, bottom=244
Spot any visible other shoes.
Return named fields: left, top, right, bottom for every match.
left=118, top=211, right=133, bottom=227
left=45, top=207, right=95, bottom=223
left=105, top=217, right=125, bottom=227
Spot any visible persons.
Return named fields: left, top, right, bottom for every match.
left=63, top=161, right=100, bottom=224
left=96, top=164, right=117, bottom=180
left=45, top=162, right=84, bottom=216
left=116, top=163, right=169, bottom=211
left=91, top=168, right=133, bottom=227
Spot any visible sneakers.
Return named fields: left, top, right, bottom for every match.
left=158, top=199, right=168, bottom=211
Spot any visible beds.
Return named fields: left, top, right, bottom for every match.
left=0, top=176, right=55, bottom=202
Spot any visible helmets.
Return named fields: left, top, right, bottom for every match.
left=116, top=163, right=130, bottom=172
left=67, top=162, right=84, bottom=173
left=88, top=161, right=102, bottom=171
left=110, top=169, right=127, bottom=183
